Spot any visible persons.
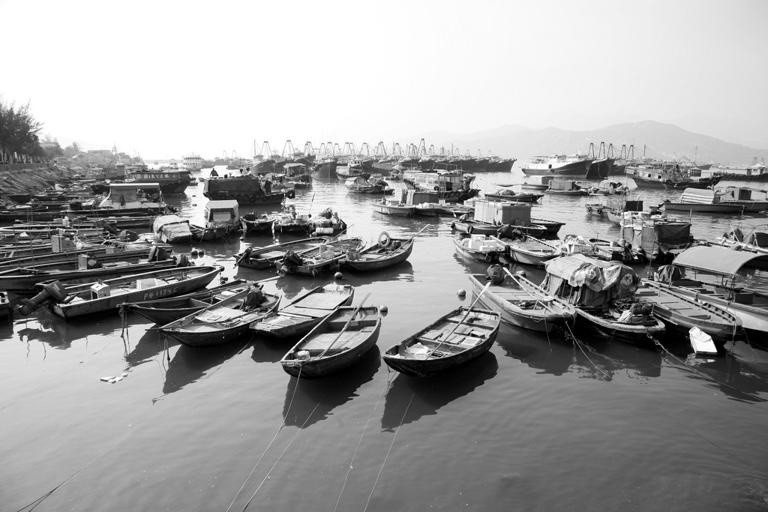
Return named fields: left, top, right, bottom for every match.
left=245, top=282, right=262, bottom=308
left=306, top=214, right=315, bottom=237
left=486, top=259, right=506, bottom=284
left=210, top=167, right=273, bottom=195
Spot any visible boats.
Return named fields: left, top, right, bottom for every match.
left=279, top=303, right=384, bottom=380
left=282, top=344, right=383, bottom=432
left=249, top=272, right=354, bottom=348
left=158, top=284, right=283, bottom=351
left=377, top=349, right=499, bottom=433
left=150, top=341, right=243, bottom=404
left=382, top=306, right=502, bottom=378
left=538, top=253, right=668, bottom=347
left=0, top=135, right=768, bottom=331
left=634, top=277, right=742, bottom=341
